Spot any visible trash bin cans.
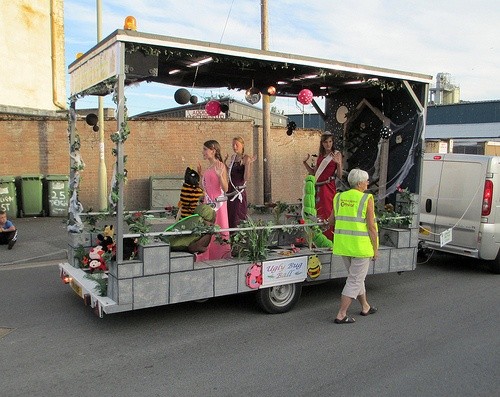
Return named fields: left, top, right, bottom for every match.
left=20, top=173, right=45, bottom=218
left=0, top=175, right=18, bottom=218
left=46, top=174, right=69, bottom=217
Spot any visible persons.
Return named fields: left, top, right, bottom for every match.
left=224, top=135, right=258, bottom=234
left=302, top=130, right=342, bottom=248
left=326, top=169, right=378, bottom=323
left=0, top=210, right=18, bottom=250
left=195, top=139, right=231, bottom=263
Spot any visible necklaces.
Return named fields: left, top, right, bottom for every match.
left=236, top=156, right=241, bottom=158
left=208, top=161, right=216, bottom=169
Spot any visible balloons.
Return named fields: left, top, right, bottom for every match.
left=86, top=113, right=100, bottom=132
left=174, top=87, right=198, bottom=104
left=297, top=88, right=313, bottom=104
left=204, top=99, right=228, bottom=116
left=379, top=126, right=393, bottom=139
left=268, top=85, right=276, bottom=102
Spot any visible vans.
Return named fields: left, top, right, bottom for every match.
left=419, top=153, right=500, bottom=275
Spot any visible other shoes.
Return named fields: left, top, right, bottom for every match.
left=334, top=314, right=356, bottom=324
left=360, top=306, right=378, bottom=316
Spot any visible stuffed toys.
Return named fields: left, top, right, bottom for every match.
left=81, top=224, right=138, bottom=271
